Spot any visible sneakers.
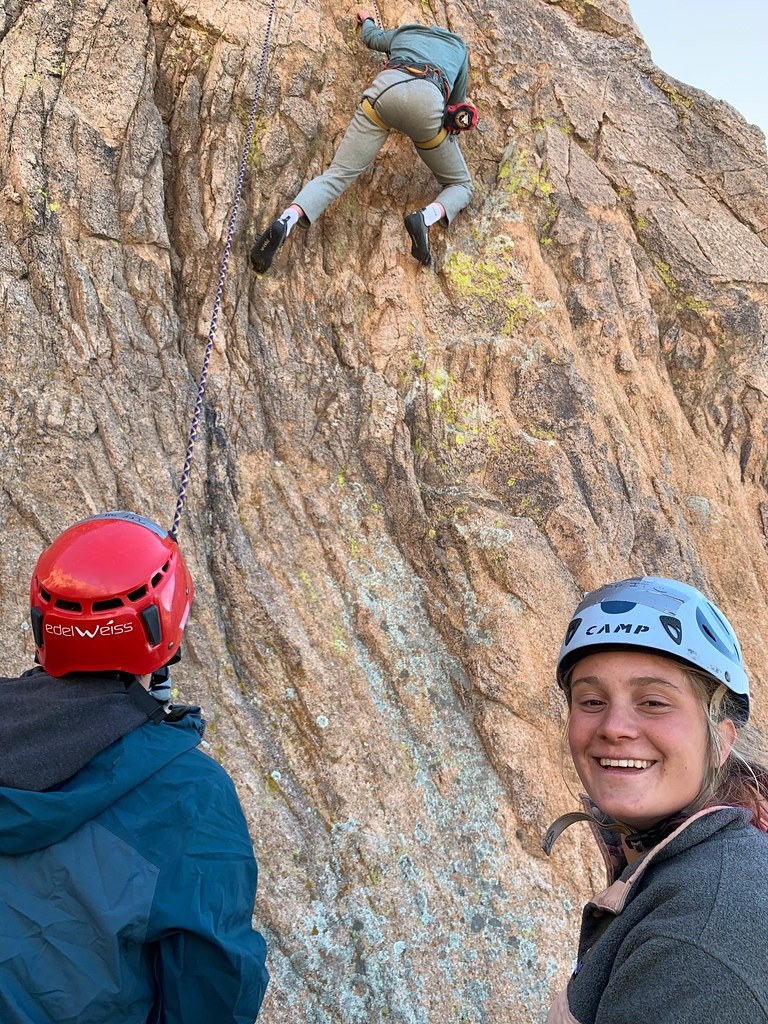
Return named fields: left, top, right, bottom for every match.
left=404, top=207, right=433, bottom=266
left=249, top=215, right=291, bottom=274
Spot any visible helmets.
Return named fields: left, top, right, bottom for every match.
left=554, top=575, right=752, bottom=730
left=29, top=509, right=196, bottom=677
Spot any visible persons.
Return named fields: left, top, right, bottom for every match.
left=543, top=574, right=768, bottom=1022
left=0, top=511, right=270, bottom=1024
left=250, top=11, right=478, bottom=275
